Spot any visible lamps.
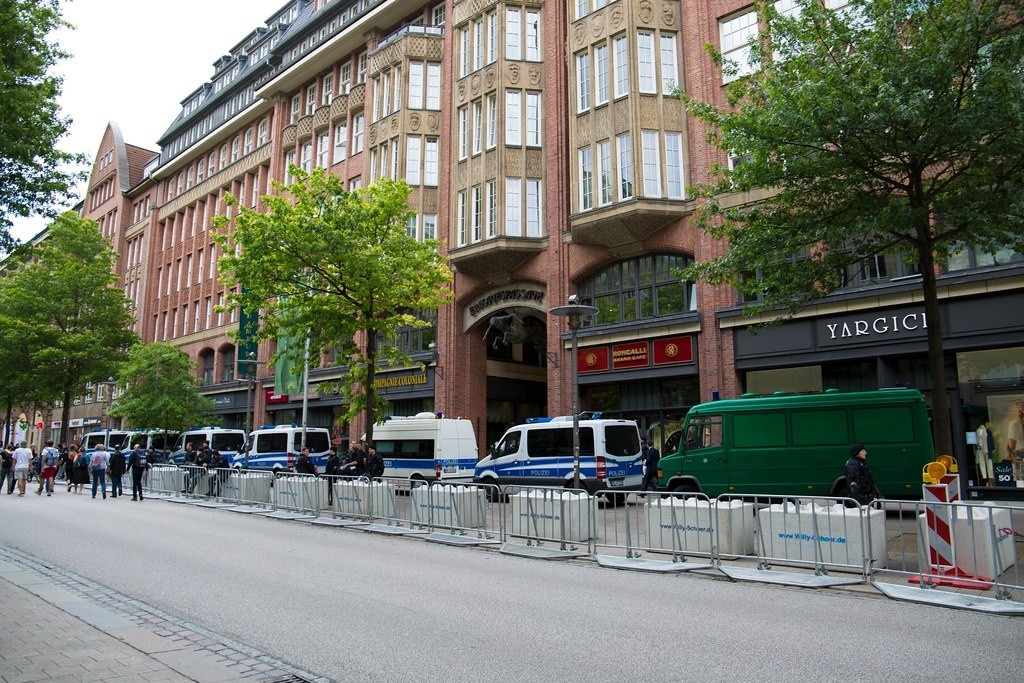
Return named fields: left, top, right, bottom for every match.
left=423, top=360, right=443, bottom=380
left=534, top=345, right=558, bottom=370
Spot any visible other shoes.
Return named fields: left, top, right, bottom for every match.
left=78, top=491, right=83, bottom=494
left=119, top=490, right=123, bottom=496
left=67, top=485, right=72, bottom=492
left=131, top=498, right=137, bottom=501
left=34, top=490, right=41, bottom=495
left=47, top=493, right=51, bottom=496
left=103, top=491, right=106, bottom=499
left=110, top=495, right=117, bottom=498
left=18, top=493, right=23, bottom=497
left=74, top=490, right=77, bottom=494
left=140, top=495, right=143, bottom=500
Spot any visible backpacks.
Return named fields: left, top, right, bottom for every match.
left=2, top=455, right=12, bottom=468
left=136, top=451, right=146, bottom=467
left=830, top=478, right=851, bottom=508
left=45, top=448, right=57, bottom=466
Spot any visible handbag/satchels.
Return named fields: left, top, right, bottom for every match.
left=643, top=465, right=647, bottom=476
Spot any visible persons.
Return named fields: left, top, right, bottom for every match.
left=364, top=446, right=384, bottom=484
left=341, top=444, right=366, bottom=482
left=109, top=444, right=126, bottom=498
left=11, top=440, right=33, bottom=497
left=0, top=440, right=19, bottom=494
left=29, top=440, right=91, bottom=496
left=295, top=447, right=321, bottom=477
left=509, top=440, right=516, bottom=449
left=89, top=444, right=111, bottom=499
left=845, top=444, right=876, bottom=508
left=146, top=446, right=157, bottom=490
left=323, top=447, right=340, bottom=505
left=1008, top=400, right=1024, bottom=461
left=126, top=444, right=147, bottom=501
left=638, top=436, right=660, bottom=498
left=181, top=440, right=232, bottom=497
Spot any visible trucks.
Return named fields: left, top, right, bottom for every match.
left=656, top=387, right=936, bottom=509
left=78, top=429, right=124, bottom=464
left=339, top=412, right=478, bottom=494
left=119, top=429, right=180, bottom=465
left=231, top=423, right=332, bottom=480
left=473, top=410, right=647, bottom=506
left=167, top=426, right=246, bottom=469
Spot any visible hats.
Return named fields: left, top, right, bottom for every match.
left=330, top=446, right=337, bottom=453
left=213, top=450, right=220, bottom=455
left=204, top=440, right=209, bottom=445
left=850, top=444, right=865, bottom=456
left=198, top=448, right=204, bottom=452
left=369, top=445, right=376, bottom=450
